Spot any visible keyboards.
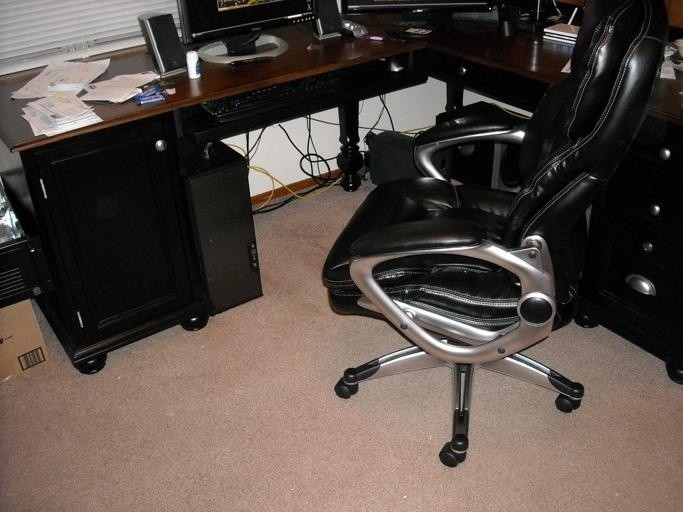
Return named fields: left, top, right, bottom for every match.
left=200, top=72, right=341, bottom=125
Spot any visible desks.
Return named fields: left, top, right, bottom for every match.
left=0, top=15, right=683, bottom=383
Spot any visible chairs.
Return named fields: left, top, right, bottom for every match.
left=323, top=1, right=667, bottom=468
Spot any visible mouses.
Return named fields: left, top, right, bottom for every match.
left=381, top=59, right=404, bottom=72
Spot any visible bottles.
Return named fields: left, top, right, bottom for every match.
left=186, top=51, right=202, bottom=79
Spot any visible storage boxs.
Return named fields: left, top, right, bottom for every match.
left=0, top=298, right=50, bottom=384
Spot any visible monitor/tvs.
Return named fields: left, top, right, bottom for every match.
left=341, top=0, right=494, bottom=39
left=177, top=0, right=320, bottom=64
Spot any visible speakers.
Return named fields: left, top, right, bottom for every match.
left=181, top=140, right=263, bottom=317
left=312, top=0, right=343, bottom=40
left=137, top=12, right=187, bottom=78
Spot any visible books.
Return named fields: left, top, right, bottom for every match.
left=11, top=58, right=160, bottom=137
